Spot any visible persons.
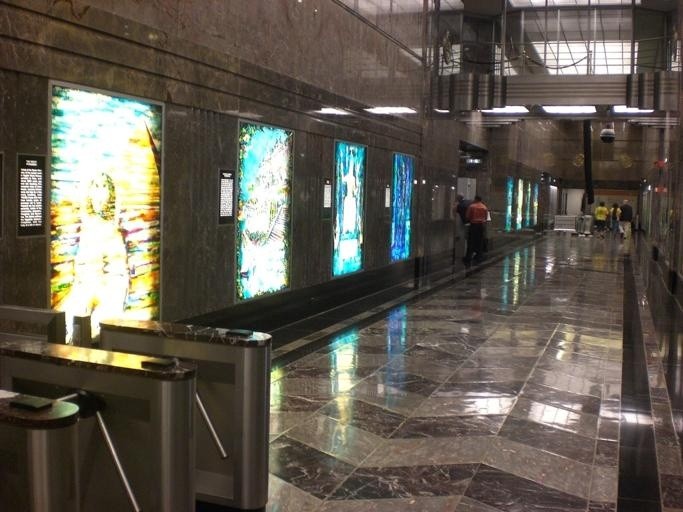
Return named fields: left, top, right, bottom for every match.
left=592, top=201, right=609, bottom=239
left=480, top=201, right=491, bottom=256
left=454, top=192, right=471, bottom=221
left=618, top=198, right=632, bottom=240
left=462, top=196, right=487, bottom=266
left=606, top=202, right=621, bottom=239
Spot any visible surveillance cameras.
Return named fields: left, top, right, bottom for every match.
left=600, top=128, right=615, bottom=143
